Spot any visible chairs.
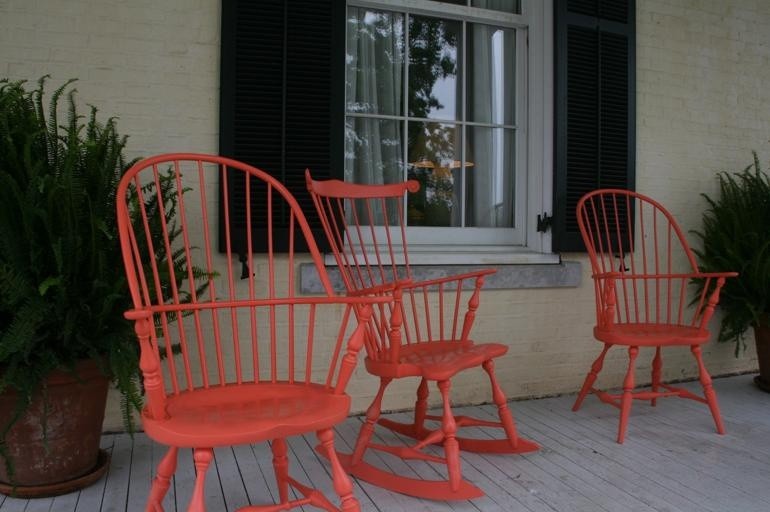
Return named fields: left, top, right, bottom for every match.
left=572, top=188, right=739, bottom=445
left=116, top=153, right=395, bottom=510
left=306, top=169, right=541, bottom=500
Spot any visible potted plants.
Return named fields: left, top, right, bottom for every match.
left=686, top=150, right=769, bottom=389
left=0, top=73, right=221, bottom=497
